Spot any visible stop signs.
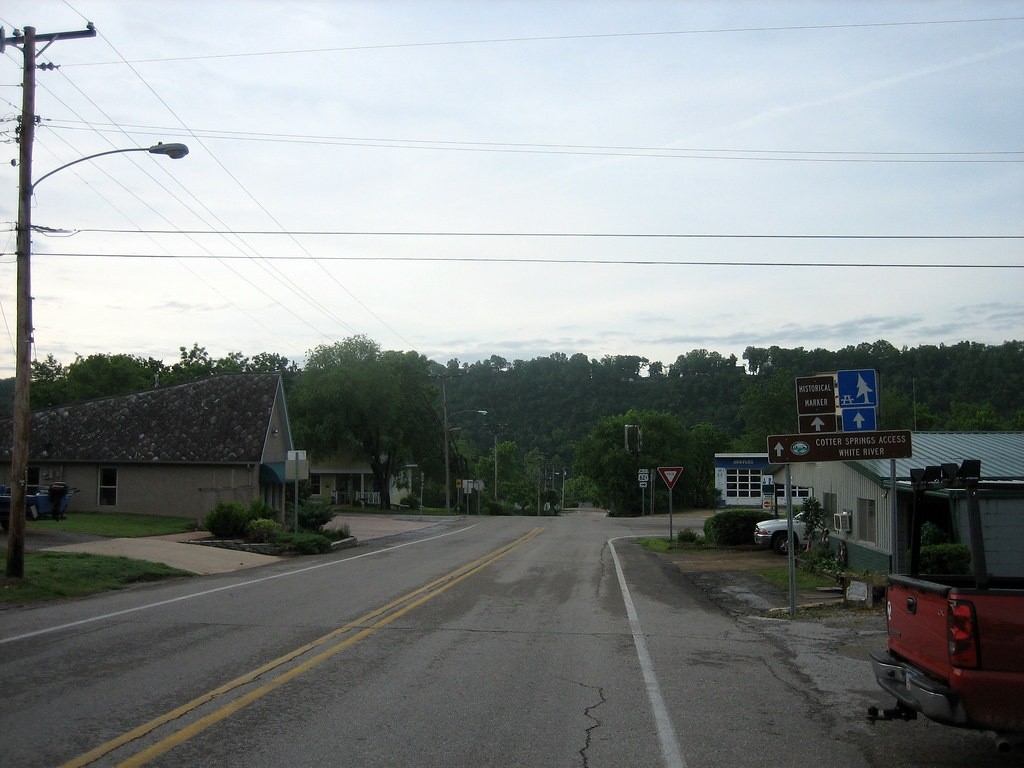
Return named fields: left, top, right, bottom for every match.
left=764, top=502, right=771, bottom=508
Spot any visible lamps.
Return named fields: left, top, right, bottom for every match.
left=274, top=425, right=278, bottom=433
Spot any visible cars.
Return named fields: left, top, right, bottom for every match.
left=754, top=511, right=811, bottom=556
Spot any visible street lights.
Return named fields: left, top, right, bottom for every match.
left=6, top=141, right=189, bottom=581
left=443, top=385, right=488, bottom=510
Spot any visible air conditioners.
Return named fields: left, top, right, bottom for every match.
left=834, top=512, right=850, bottom=531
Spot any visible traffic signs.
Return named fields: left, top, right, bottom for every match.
left=766, top=369, right=912, bottom=464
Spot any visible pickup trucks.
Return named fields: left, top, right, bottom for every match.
left=866, top=459, right=1024, bottom=757
